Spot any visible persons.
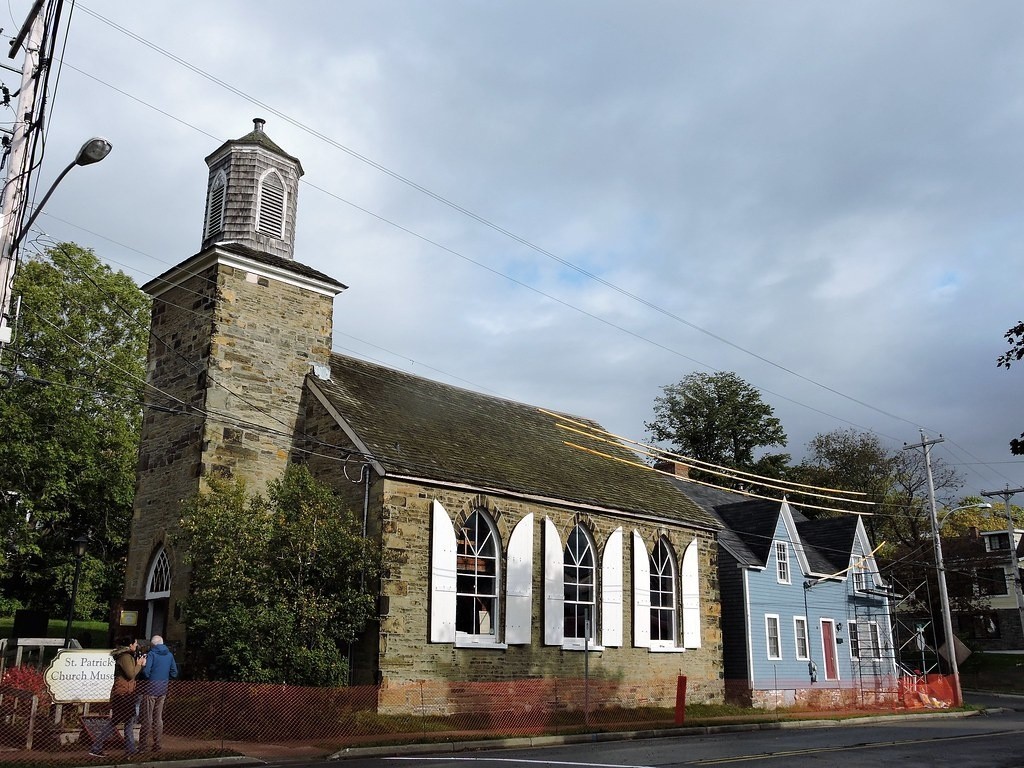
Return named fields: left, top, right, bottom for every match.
left=87, top=639, right=148, bottom=760
left=140, top=636, right=178, bottom=754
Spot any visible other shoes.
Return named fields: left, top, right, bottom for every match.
left=89, top=746, right=110, bottom=758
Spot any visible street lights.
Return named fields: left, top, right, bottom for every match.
left=48, top=533, right=92, bottom=723
left=0, top=137, right=114, bottom=347
left=931, top=502, right=992, bottom=710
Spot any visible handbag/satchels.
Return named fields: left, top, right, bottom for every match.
left=112, top=690, right=136, bottom=723
